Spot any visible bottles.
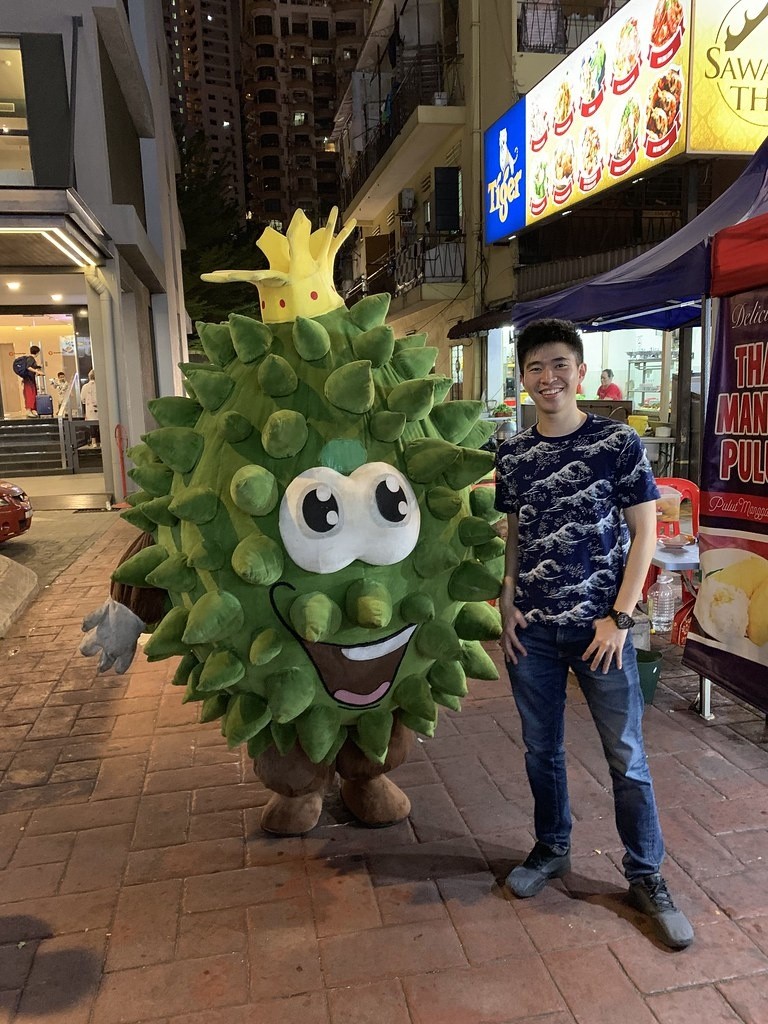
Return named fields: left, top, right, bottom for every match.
left=647, top=576, right=675, bottom=631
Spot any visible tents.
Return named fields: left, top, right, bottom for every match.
left=506, top=134, right=767, bottom=433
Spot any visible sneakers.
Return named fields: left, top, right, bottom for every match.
left=26, top=412, right=37, bottom=418
left=626, top=874, right=696, bottom=950
left=504, top=842, right=571, bottom=898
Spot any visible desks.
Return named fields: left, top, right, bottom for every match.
left=639, top=434, right=676, bottom=477
left=650, top=537, right=699, bottom=598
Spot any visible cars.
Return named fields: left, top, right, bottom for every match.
left=0, top=479, right=34, bottom=543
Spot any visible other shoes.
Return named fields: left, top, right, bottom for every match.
left=92, top=443, right=97, bottom=448
left=56, top=412, right=59, bottom=415
left=65, top=413, right=68, bottom=417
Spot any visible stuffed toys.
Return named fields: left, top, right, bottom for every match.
left=82, top=204, right=507, bottom=834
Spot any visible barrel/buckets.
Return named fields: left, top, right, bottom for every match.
left=628, top=415, right=648, bottom=436
left=635, top=648, right=662, bottom=704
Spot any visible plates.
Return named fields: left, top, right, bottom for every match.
left=659, top=540, right=690, bottom=548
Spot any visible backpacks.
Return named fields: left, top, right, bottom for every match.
left=13, top=356, right=35, bottom=379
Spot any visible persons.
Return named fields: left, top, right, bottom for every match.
left=494, top=318, right=695, bottom=948
left=22, top=346, right=102, bottom=448
left=593, top=369, right=622, bottom=400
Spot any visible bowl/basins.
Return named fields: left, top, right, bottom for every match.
left=505, top=400, right=516, bottom=406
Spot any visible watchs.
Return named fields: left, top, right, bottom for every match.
left=609, top=608, right=635, bottom=629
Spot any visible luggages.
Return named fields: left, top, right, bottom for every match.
left=35, top=374, right=54, bottom=418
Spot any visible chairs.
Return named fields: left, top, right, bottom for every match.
left=641, top=478, right=700, bottom=605
